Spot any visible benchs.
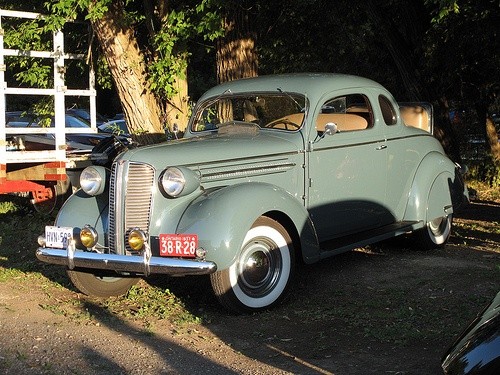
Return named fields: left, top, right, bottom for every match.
left=264, top=111, right=371, bottom=133
left=341, top=100, right=434, bottom=136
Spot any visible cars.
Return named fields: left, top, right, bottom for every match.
left=35, top=71, right=474, bottom=313
left=5, top=109, right=129, bottom=148
